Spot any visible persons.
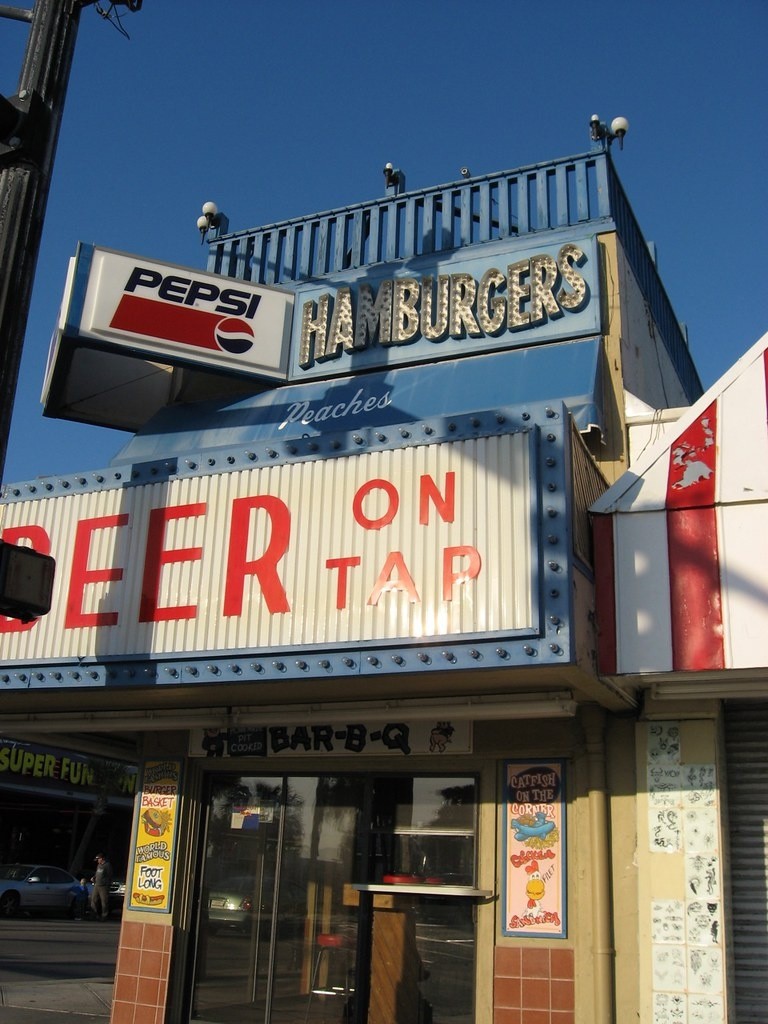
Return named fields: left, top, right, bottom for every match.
left=90, top=853, right=113, bottom=921
left=70, top=877, right=89, bottom=921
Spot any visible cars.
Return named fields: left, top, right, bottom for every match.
left=204, top=876, right=309, bottom=936
left=0, top=864, right=126, bottom=917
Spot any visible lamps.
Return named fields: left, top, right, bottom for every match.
left=196, top=202, right=219, bottom=245
left=605, top=116, right=630, bottom=150
left=382, top=163, right=398, bottom=189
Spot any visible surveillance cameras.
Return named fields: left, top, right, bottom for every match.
left=460, top=167, right=470, bottom=178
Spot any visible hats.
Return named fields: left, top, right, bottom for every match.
left=94, top=853, right=103, bottom=861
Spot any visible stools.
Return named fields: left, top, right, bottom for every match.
left=304, top=934, right=354, bottom=1024
